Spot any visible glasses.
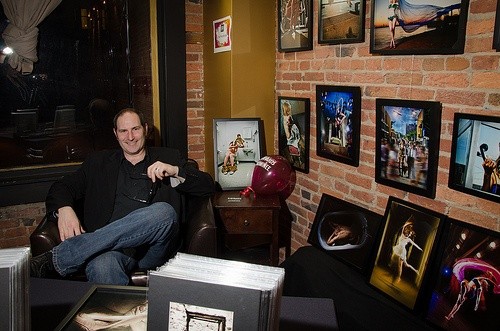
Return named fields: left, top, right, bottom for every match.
left=121, top=173, right=158, bottom=204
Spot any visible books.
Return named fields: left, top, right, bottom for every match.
left=0, top=245, right=35, bottom=331
left=148, top=250, right=286, bottom=330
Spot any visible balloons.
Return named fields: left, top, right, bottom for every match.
left=251, top=154, right=293, bottom=196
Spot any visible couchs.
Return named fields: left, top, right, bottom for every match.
left=30, top=197, right=218, bottom=287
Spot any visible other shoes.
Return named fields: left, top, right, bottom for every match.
left=32, top=250, right=55, bottom=287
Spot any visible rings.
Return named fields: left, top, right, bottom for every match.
left=154, top=167, right=159, bottom=171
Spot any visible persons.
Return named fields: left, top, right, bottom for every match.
left=444, top=276, right=498, bottom=321
left=480, top=142, right=500, bottom=196
left=389, top=219, right=424, bottom=288
left=388, top=0, right=397, bottom=50
left=327, top=220, right=352, bottom=245
left=28, top=107, right=215, bottom=287
left=321, top=94, right=353, bottom=154
left=281, top=101, right=303, bottom=168
left=381, top=136, right=429, bottom=186
left=221, top=134, right=247, bottom=176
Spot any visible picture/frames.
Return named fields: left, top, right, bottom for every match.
left=366, top=196, right=448, bottom=314
left=307, top=193, right=385, bottom=277
left=369, top=0, right=469, bottom=56
left=375, top=98, right=440, bottom=200
left=278, top=96, right=311, bottom=174
left=278, top=0, right=313, bottom=53
left=421, top=218, right=500, bottom=331
left=55, top=285, right=149, bottom=331
left=316, top=85, right=360, bottom=167
left=318, top=1, right=365, bottom=44
left=448, top=113, right=500, bottom=204
left=213, top=118, right=264, bottom=191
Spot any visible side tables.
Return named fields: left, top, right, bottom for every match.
left=211, top=191, right=281, bottom=267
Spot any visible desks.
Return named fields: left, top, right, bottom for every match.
left=30, top=278, right=338, bottom=331
